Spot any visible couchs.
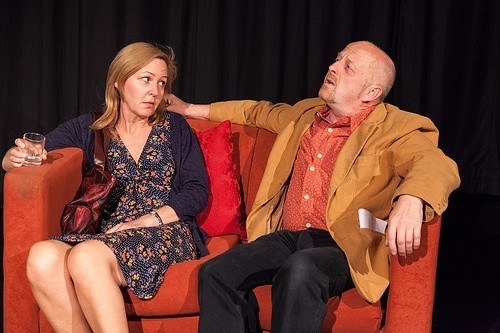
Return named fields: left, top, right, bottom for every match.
left=3, top=118, right=444, bottom=333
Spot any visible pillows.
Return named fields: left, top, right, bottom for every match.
left=196, top=120, right=248, bottom=241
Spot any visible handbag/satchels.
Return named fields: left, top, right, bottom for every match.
left=60, top=106, right=116, bottom=236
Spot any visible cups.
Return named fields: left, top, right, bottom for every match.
left=23, top=131, right=46, bottom=166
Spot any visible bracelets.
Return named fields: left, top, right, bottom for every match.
left=149, top=210, right=166, bottom=228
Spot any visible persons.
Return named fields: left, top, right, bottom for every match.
left=164, top=40, right=462, bottom=333
left=2, top=42, right=210, bottom=333
